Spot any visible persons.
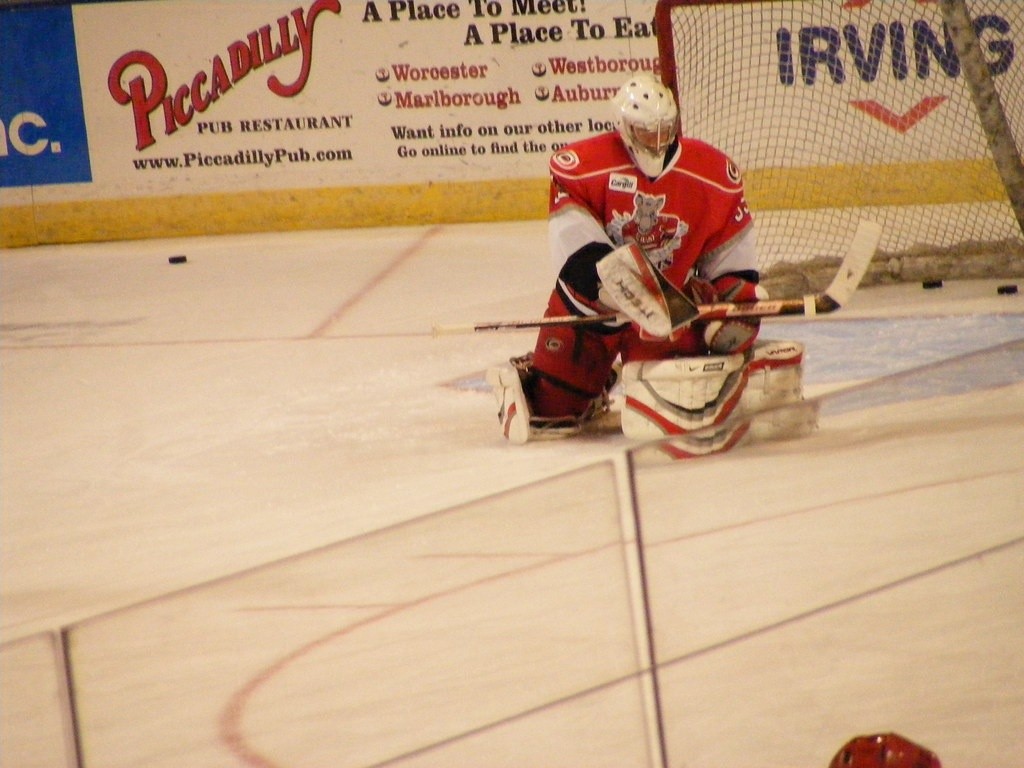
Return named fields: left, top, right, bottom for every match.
left=528, top=72, right=770, bottom=420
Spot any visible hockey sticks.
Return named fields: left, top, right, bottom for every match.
left=427, top=215, right=887, bottom=341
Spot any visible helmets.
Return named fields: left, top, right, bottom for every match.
left=612, top=75, right=677, bottom=178
left=828, top=732, right=945, bottom=768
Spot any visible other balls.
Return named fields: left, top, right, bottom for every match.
left=169, top=255, right=187, bottom=264
left=996, top=285, right=1018, bottom=294
left=922, top=278, right=943, bottom=289
